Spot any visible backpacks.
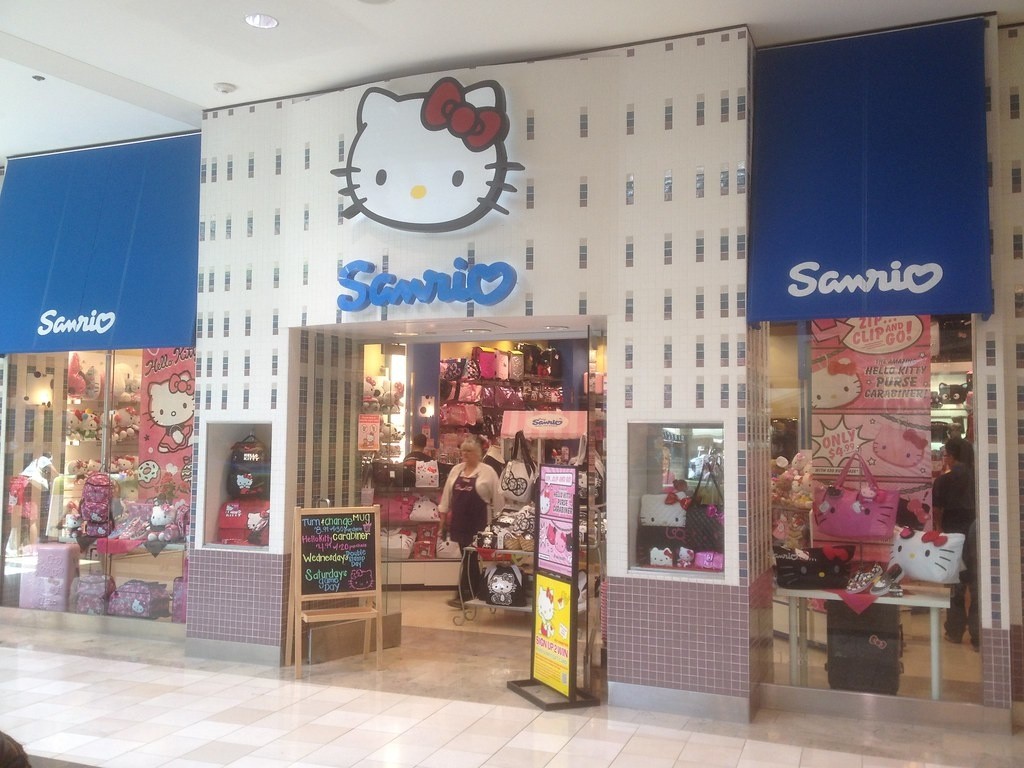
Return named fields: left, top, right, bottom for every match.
left=124, top=498, right=190, bottom=541
left=219, top=501, right=270, bottom=545
left=227, top=435, right=270, bottom=500
left=81, top=464, right=120, bottom=523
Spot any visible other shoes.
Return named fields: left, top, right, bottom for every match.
left=869, top=563, right=905, bottom=597
left=846, top=562, right=883, bottom=593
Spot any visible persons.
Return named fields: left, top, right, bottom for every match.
left=933, top=438, right=979, bottom=652
left=402, top=433, right=435, bottom=462
left=5, top=451, right=61, bottom=558
left=436, top=437, right=505, bottom=608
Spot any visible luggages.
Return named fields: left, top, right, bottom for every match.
left=20, top=543, right=79, bottom=612
left=824, top=600, right=904, bottom=695
left=68, top=547, right=116, bottom=615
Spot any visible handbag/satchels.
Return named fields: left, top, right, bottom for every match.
left=772, top=546, right=856, bottom=590
left=85, top=516, right=114, bottom=538
left=172, top=577, right=183, bottom=622
left=476, top=564, right=527, bottom=607
left=370, top=343, right=564, bottom=552
left=684, top=463, right=724, bottom=552
left=812, top=452, right=899, bottom=540
left=108, top=579, right=170, bottom=620
